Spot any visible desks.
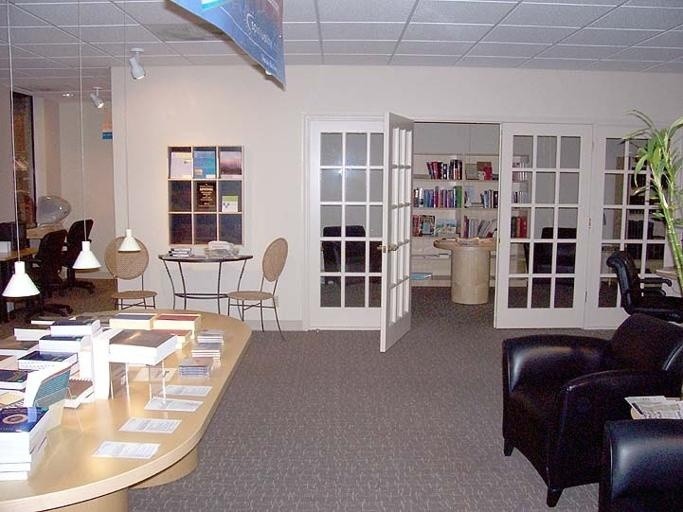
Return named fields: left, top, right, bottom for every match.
left=1, top=247, right=39, bottom=319
left=0, top=311, right=251, bottom=511
left=159, top=253, right=252, bottom=317
left=432, top=239, right=497, bottom=306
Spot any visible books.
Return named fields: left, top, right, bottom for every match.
left=465, top=214, right=527, bottom=239
left=170, top=151, right=192, bottom=181
left=193, top=151, right=216, bottom=180
left=205, top=239, right=237, bottom=262
left=101, top=327, right=178, bottom=366
left=13, top=325, right=50, bottom=342
left=110, top=311, right=156, bottom=331
left=413, top=185, right=462, bottom=208
left=38, top=335, right=91, bottom=372
left=219, top=150, right=243, bottom=179
left=179, top=357, right=215, bottom=377
left=150, top=328, right=191, bottom=350
left=426, top=159, right=463, bottom=180
left=465, top=162, right=477, bottom=178
left=17, top=351, right=80, bottom=380
left=155, top=312, right=202, bottom=339
left=0, top=367, right=93, bottom=429
left=413, top=213, right=458, bottom=237
left=49, top=317, right=110, bottom=367
left=191, top=344, right=223, bottom=360
left=479, top=189, right=517, bottom=207
left=475, top=161, right=492, bottom=180
left=0, top=408, right=48, bottom=480
left=221, top=195, right=238, bottom=213
left=199, top=328, right=225, bottom=345
left=161, top=244, right=197, bottom=260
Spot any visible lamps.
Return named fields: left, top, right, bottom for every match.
left=1, top=0, right=39, bottom=298
left=89, top=86, right=105, bottom=108
left=115, top=1, right=142, bottom=253
left=128, top=47, right=146, bottom=80
left=70, top=1, right=101, bottom=270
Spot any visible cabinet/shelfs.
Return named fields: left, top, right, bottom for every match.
left=168, top=146, right=244, bottom=246
left=411, top=153, right=530, bottom=288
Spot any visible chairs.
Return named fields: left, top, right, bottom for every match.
left=606, top=251, right=683, bottom=321
left=103, top=237, right=156, bottom=311
left=502, top=312, right=682, bottom=507
left=9, top=230, right=73, bottom=325
left=523, top=227, right=576, bottom=286
left=322, top=226, right=383, bottom=290
left=227, top=238, right=288, bottom=340
left=34, top=219, right=96, bottom=296
left=598, top=418, right=682, bottom=510
